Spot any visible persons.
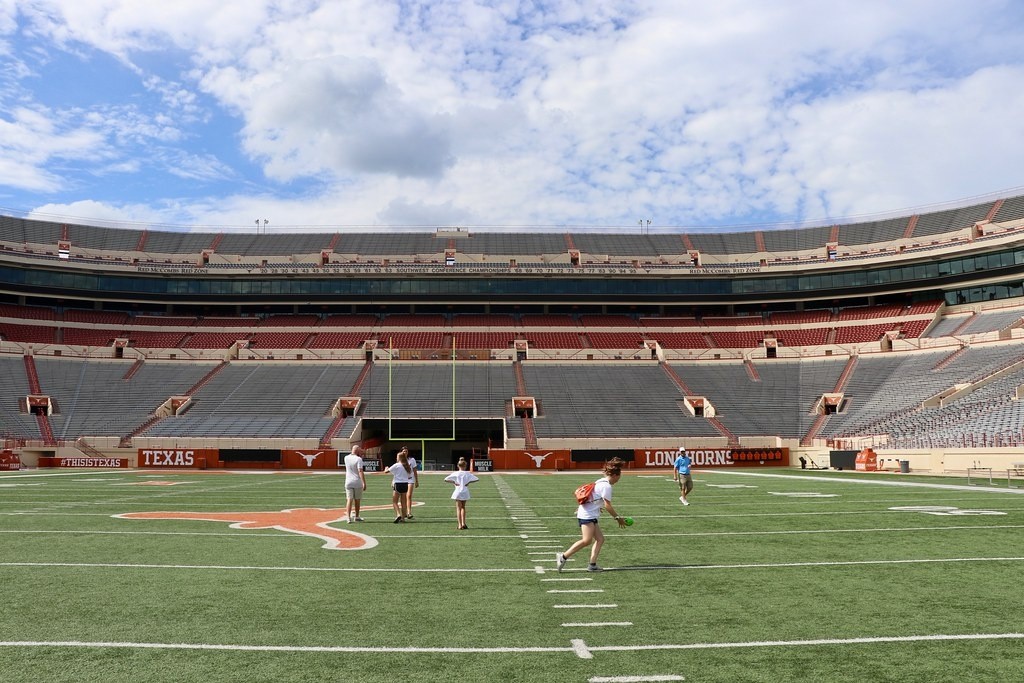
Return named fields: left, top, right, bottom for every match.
left=674, top=447, right=693, bottom=506
left=556, top=457, right=628, bottom=573
left=444, top=457, right=479, bottom=529
left=385, top=452, right=411, bottom=523
left=344, top=445, right=366, bottom=523
left=400, top=446, right=418, bottom=518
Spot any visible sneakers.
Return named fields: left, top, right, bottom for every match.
left=679, top=496, right=683, bottom=501
left=587, top=564, right=603, bottom=572
left=682, top=500, right=690, bottom=506
left=347, top=520, right=351, bottom=524
left=556, top=552, right=566, bottom=573
left=354, top=517, right=364, bottom=521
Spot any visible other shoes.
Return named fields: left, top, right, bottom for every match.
left=463, top=525, right=467, bottom=529
left=457, top=527, right=463, bottom=529
left=407, top=513, right=413, bottom=519
left=394, top=515, right=401, bottom=523
left=399, top=518, right=405, bottom=522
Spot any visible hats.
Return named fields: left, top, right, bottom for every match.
left=679, top=447, right=685, bottom=451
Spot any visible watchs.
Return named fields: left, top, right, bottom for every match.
left=615, top=516, right=619, bottom=520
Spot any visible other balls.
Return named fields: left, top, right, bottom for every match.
left=624, top=517, right=633, bottom=526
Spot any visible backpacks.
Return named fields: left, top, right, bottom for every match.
left=574, top=481, right=607, bottom=505
